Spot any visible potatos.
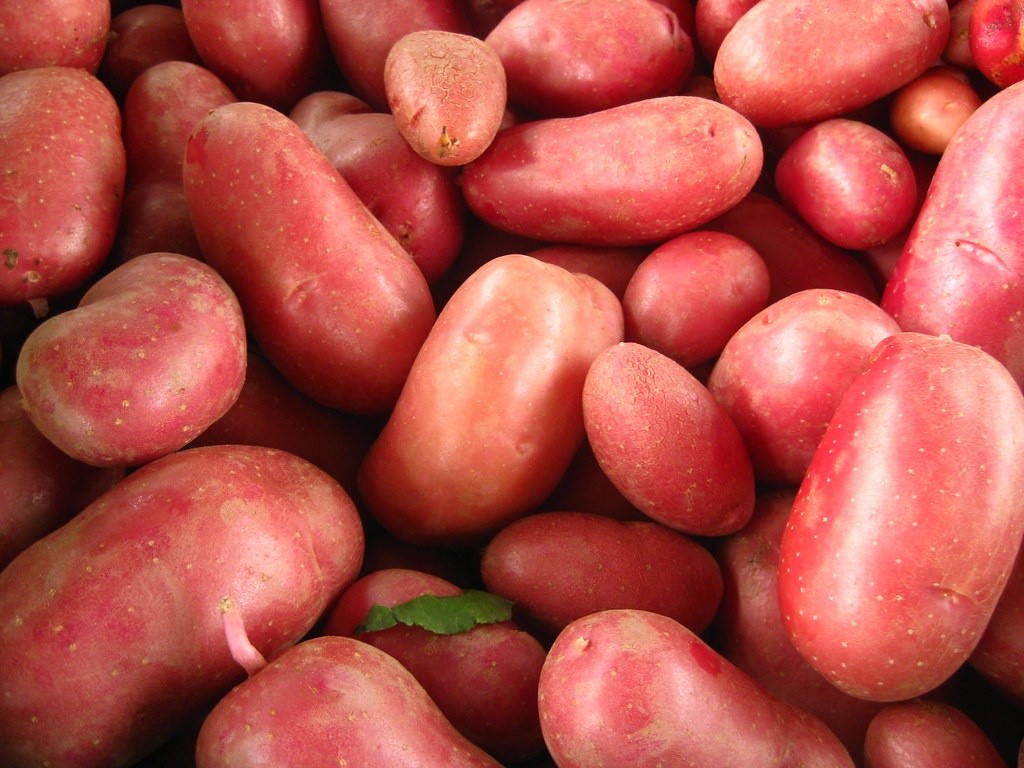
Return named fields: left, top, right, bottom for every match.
left=0, top=0, right=1024, bottom=768
